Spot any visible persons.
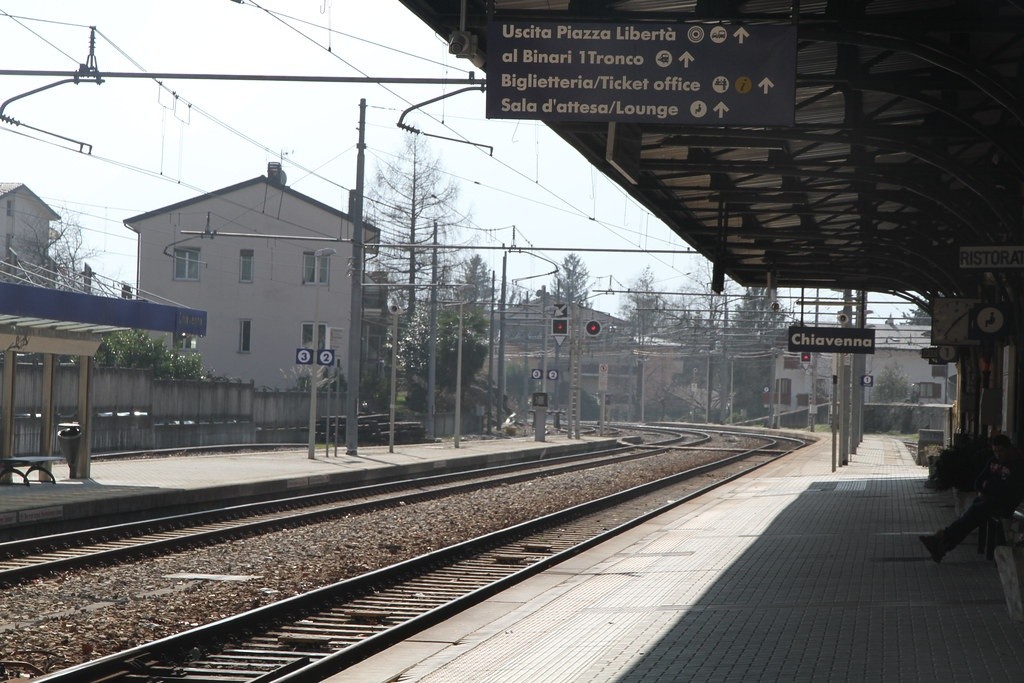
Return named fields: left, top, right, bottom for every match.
left=919, top=434, right=1024, bottom=562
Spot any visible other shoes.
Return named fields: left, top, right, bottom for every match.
left=920, top=530, right=953, bottom=564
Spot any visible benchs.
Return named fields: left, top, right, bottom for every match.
left=4, top=456, right=63, bottom=484
left=977, top=508, right=1024, bottom=561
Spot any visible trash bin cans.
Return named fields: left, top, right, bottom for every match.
left=57, top=423, right=82, bottom=467
